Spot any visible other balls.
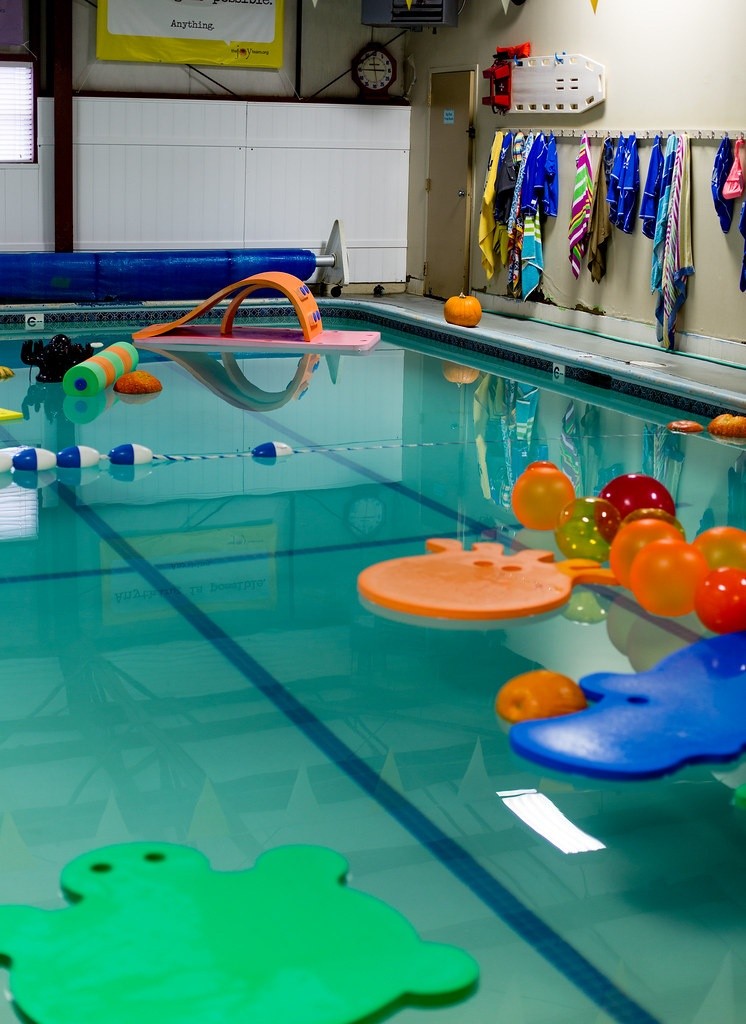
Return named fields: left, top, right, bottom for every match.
left=513, top=457, right=746, bottom=632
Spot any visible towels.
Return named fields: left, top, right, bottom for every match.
left=738, top=199, right=746, bottom=292
left=478, top=130, right=559, bottom=300
left=568, top=133, right=594, bottom=281
left=640, top=135, right=696, bottom=350
left=711, top=135, right=746, bottom=234
left=588, top=132, right=612, bottom=285
left=603, top=132, right=640, bottom=235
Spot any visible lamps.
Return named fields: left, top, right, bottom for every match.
left=343, top=486, right=387, bottom=537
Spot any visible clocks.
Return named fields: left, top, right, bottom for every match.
left=351, top=42, right=397, bottom=96
